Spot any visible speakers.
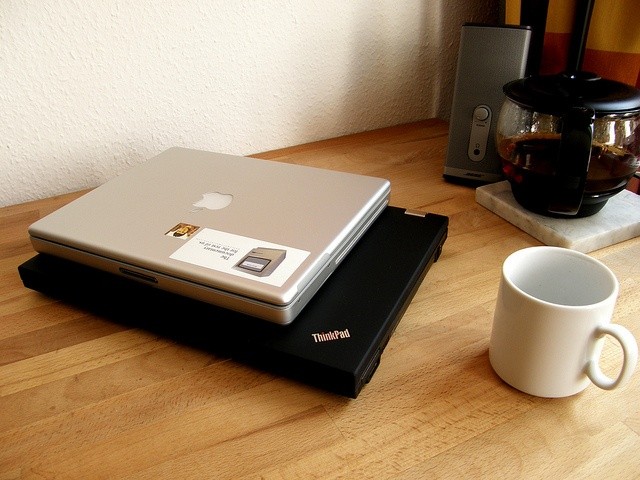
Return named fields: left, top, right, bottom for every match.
left=442, top=22, right=533, bottom=188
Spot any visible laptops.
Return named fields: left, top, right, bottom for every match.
left=18, top=206, right=449, bottom=399
left=28, top=146, right=392, bottom=327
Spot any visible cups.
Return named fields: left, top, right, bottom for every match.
left=490, top=246, right=637, bottom=399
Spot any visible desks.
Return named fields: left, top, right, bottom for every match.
left=0, top=118, right=640, bottom=479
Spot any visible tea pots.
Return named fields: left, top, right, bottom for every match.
left=499, top=67, right=640, bottom=220
